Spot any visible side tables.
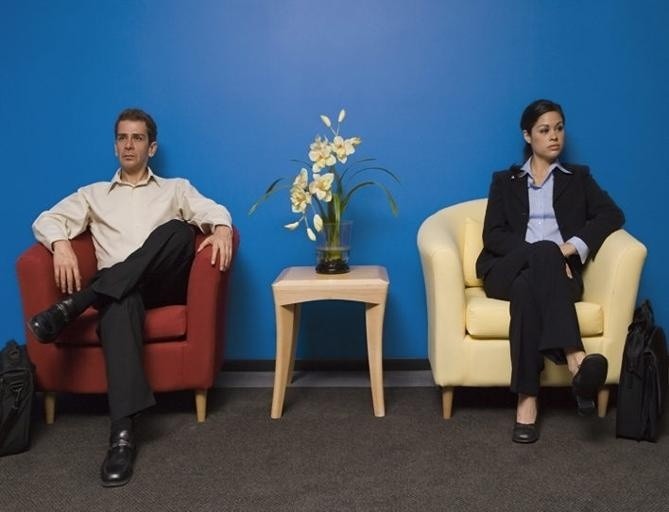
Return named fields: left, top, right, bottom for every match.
left=269, top=263, right=393, bottom=419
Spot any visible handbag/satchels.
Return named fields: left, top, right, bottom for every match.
left=1, top=340, right=36, bottom=455
left=617, top=301, right=668, bottom=443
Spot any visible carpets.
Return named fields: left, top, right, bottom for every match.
left=0, top=385, right=668, bottom=511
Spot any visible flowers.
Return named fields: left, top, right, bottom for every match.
left=247, top=109, right=402, bottom=262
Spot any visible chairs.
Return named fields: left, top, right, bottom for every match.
left=415, top=196, right=648, bottom=422
left=16, top=227, right=240, bottom=424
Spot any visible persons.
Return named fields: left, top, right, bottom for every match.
left=28, top=107, right=235, bottom=488
left=474, top=99, right=626, bottom=446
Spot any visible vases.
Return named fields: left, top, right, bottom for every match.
left=314, top=222, right=350, bottom=274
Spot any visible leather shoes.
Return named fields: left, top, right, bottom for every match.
left=30, top=300, right=68, bottom=344
left=99, top=430, right=135, bottom=487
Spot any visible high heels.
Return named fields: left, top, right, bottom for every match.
left=570, top=353, right=608, bottom=418
left=512, top=394, right=544, bottom=443
